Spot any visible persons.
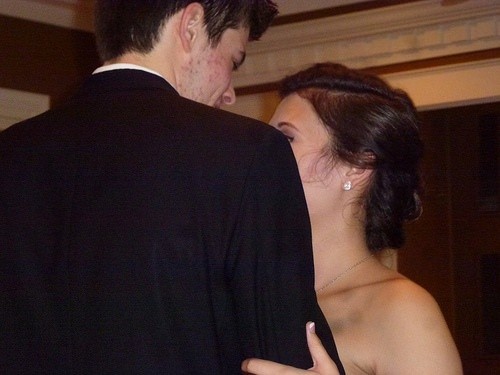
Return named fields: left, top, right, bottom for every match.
left=0, top=0, right=346, bottom=375
left=240, top=61, right=467, bottom=375
